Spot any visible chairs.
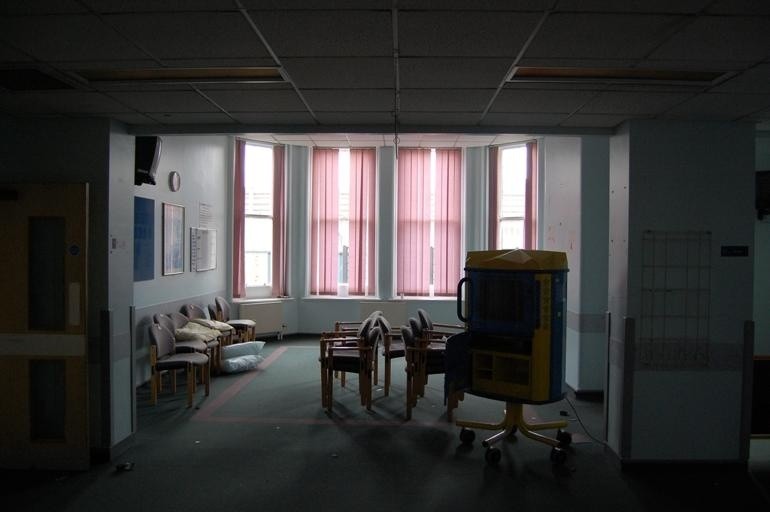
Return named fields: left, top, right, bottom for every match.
left=146, top=296, right=256, bottom=408
left=320, top=308, right=463, bottom=420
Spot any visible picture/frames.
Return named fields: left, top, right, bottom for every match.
left=162, top=201, right=185, bottom=276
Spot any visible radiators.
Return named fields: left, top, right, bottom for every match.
left=359, top=301, right=409, bottom=336
left=237, top=301, right=286, bottom=341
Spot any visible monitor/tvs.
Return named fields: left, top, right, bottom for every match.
left=135, top=136, right=164, bottom=180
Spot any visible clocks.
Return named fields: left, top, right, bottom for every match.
left=169, top=170, right=181, bottom=192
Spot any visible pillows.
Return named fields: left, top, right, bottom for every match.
left=221, top=355, right=264, bottom=375
left=221, top=341, right=266, bottom=360
